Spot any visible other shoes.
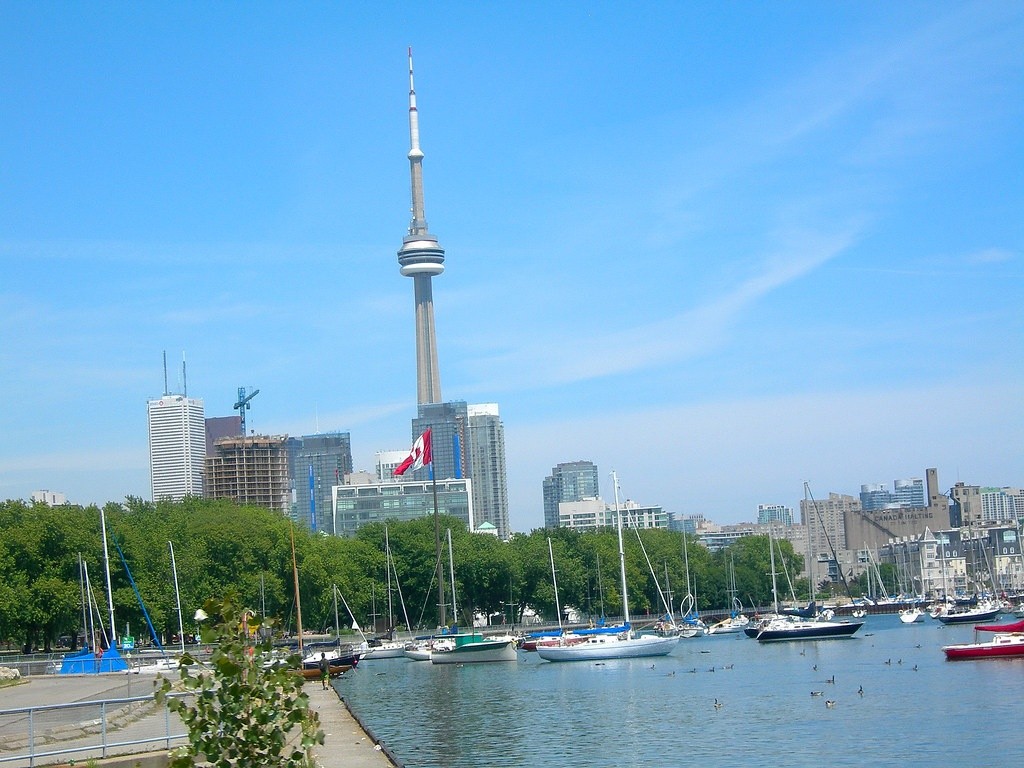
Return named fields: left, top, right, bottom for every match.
left=326, top=686, right=329, bottom=690
left=322, top=686, right=325, bottom=690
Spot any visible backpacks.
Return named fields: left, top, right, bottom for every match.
left=319, top=659, right=326, bottom=671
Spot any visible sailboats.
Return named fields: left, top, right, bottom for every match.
left=45, top=509, right=214, bottom=673
left=755, top=482, right=866, bottom=643
left=535, top=470, right=684, bottom=662
left=850, top=494, right=1024, bottom=625
left=651, top=517, right=835, bottom=639
left=264, top=516, right=624, bottom=680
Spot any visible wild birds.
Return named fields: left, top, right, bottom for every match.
left=800, top=644, right=922, bottom=683
left=857, top=685, right=864, bottom=696
left=810, top=691, right=823, bottom=695
left=825, top=700, right=836, bottom=705
left=714, top=699, right=722, bottom=707
left=650, top=664, right=734, bottom=676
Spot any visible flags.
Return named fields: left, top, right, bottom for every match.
left=393, top=427, right=432, bottom=476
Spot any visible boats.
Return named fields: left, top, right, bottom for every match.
left=941, top=620, right=1024, bottom=658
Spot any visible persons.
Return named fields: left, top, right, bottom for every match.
left=95, top=645, right=103, bottom=661
left=318, top=652, right=330, bottom=690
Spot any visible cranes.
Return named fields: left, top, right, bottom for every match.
left=233, top=387, right=260, bottom=438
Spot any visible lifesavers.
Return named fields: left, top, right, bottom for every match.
left=96, top=648, right=103, bottom=658
left=1020, top=607, right=1024, bottom=611
left=899, top=610, right=903, bottom=614
left=678, top=625, right=683, bottom=630
